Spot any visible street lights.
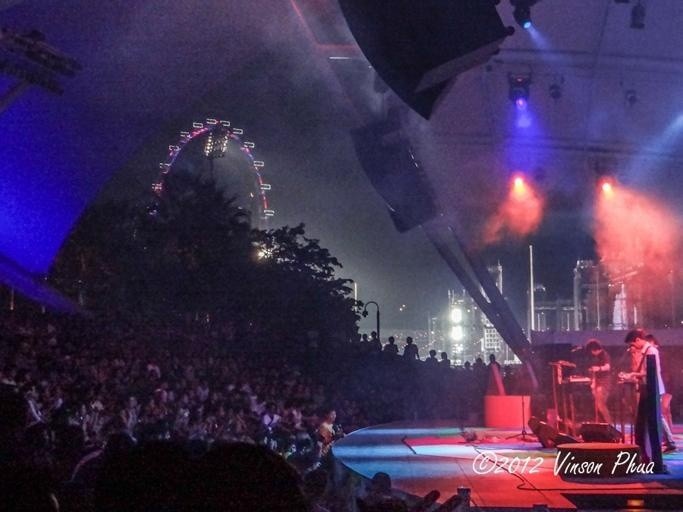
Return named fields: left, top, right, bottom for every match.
left=361, top=300, right=380, bottom=339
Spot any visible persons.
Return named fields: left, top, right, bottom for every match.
left=616, top=329, right=679, bottom=455
left=643, top=335, right=674, bottom=444
left=1, top=315, right=522, bottom=511
left=584, top=339, right=614, bottom=423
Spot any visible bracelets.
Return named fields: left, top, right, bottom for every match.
left=598, top=366, right=601, bottom=371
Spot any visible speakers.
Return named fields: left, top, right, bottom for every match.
left=347, top=117, right=441, bottom=232
left=338, top=1, right=508, bottom=117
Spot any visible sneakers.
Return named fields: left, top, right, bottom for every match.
left=664, top=445, right=678, bottom=453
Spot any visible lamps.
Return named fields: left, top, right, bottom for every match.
left=510, top=1, right=537, bottom=30
left=505, top=71, right=533, bottom=113
